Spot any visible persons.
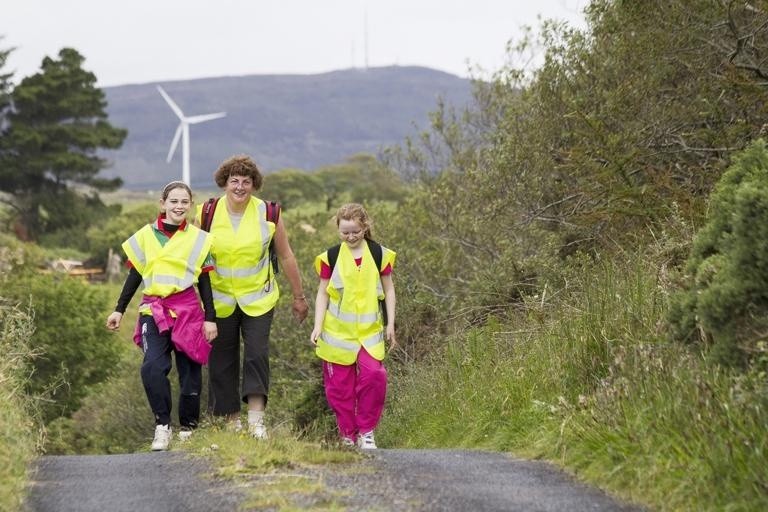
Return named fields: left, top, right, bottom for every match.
left=193, top=155, right=309, bottom=440
left=106, top=181, right=218, bottom=452
left=310, top=203, right=397, bottom=450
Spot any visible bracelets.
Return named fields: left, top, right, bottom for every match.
left=294, top=296, right=305, bottom=300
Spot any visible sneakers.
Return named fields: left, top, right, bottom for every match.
left=235, top=420, right=242, bottom=431
left=357, top=431, right=377, bottom=451
left=150, top=423, right=172, bottom=452
left=247, top=409, right=269, bottom=442
left=341, top=438, right=354, bottom=449
left=178, top=429, right=194, bottom=444
